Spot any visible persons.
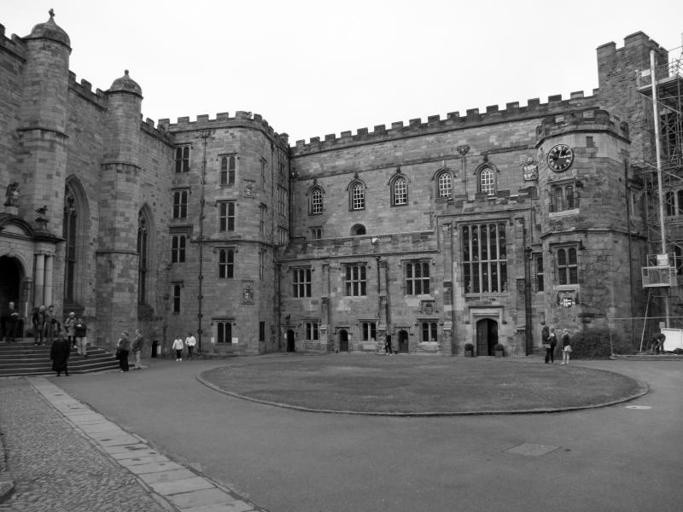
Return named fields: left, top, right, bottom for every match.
left=48, top=332, right=71, bottom=377
left=383, top=332, right=393, bottom=357
left=183, top=332, right=197, bottom=360
left=130, top=328, right=144, bottom=372
left=30, top=303, right=89, bottom=359
left=558, top=328, right=573, bottom=367
left=170, top=334, right=183, bottom=362
left=3, top=301, right=19, bottom=344
left=649, top=332, right=666, bottom=355
left=543, top=327, right=557, bottom=364
left=112, top=327, right=131, bottom=373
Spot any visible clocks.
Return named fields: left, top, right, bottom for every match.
left=546, top=142, right=574, bottom=174
